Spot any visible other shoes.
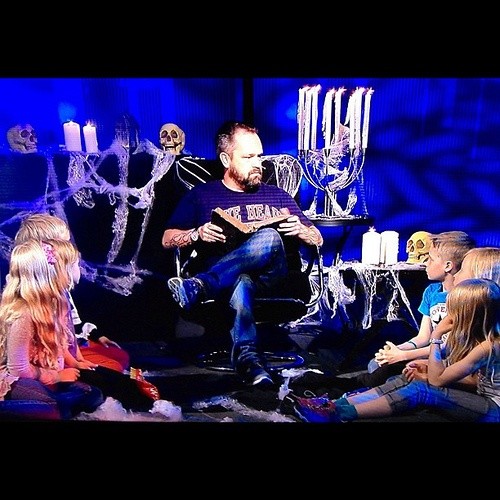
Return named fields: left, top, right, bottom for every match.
left=281, top=371, right=334, bottom=414
left=293, top=393, right=337, bottom=423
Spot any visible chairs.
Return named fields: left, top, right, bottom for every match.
left=173, top=153, right=324, bottom=373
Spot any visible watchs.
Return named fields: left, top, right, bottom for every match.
left=190, top=228, right=201, bottom=243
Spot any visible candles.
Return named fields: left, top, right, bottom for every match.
left=360, top=226, right=400, bottom=265
left=63, top=119, right=98, bottom=153
left=296, top=84, right=374, bottom=150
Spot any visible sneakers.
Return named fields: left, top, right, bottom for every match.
left=167, top=277, right=202, bottom=311
left=237, top=360, right=274, bottom=386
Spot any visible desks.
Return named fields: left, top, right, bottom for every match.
left=309, top=217, right=375, bottom=264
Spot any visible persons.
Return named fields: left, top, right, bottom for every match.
left=286, top=278, right=500, bottom=422
left=0, top=215, right=158, bottom=420
left=293, top=231, right=500, bottom=399
left=161, top=120, right=323, bottom=389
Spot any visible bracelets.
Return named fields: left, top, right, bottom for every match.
left=430, top=338, right=443, bottom=344
left=407, top=340, right=417, bottom=349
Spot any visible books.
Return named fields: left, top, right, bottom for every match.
left=210, top=207, right=299, bottom=253
